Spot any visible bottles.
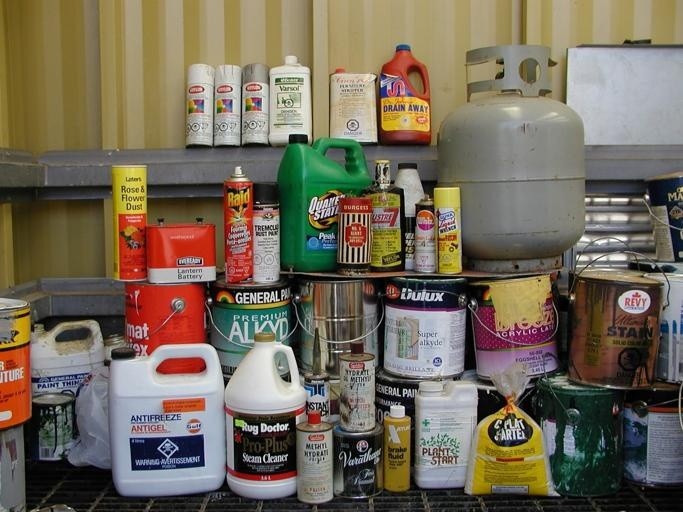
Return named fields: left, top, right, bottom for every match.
left=359, top=155, right=463, bottom=275
left=295, top=411, right=335, bottom=506
left=221, top=165, right=280, bottom=285
left=381, top=404, right=413, bottom=492
left=184, top=55, right=313, bottom=147
left=303, top=326, right=329, bottom=419
left=329, top=66, right=379, bottom=148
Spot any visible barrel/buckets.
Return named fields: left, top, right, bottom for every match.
left=1, top=45, right=682, bottom=499
